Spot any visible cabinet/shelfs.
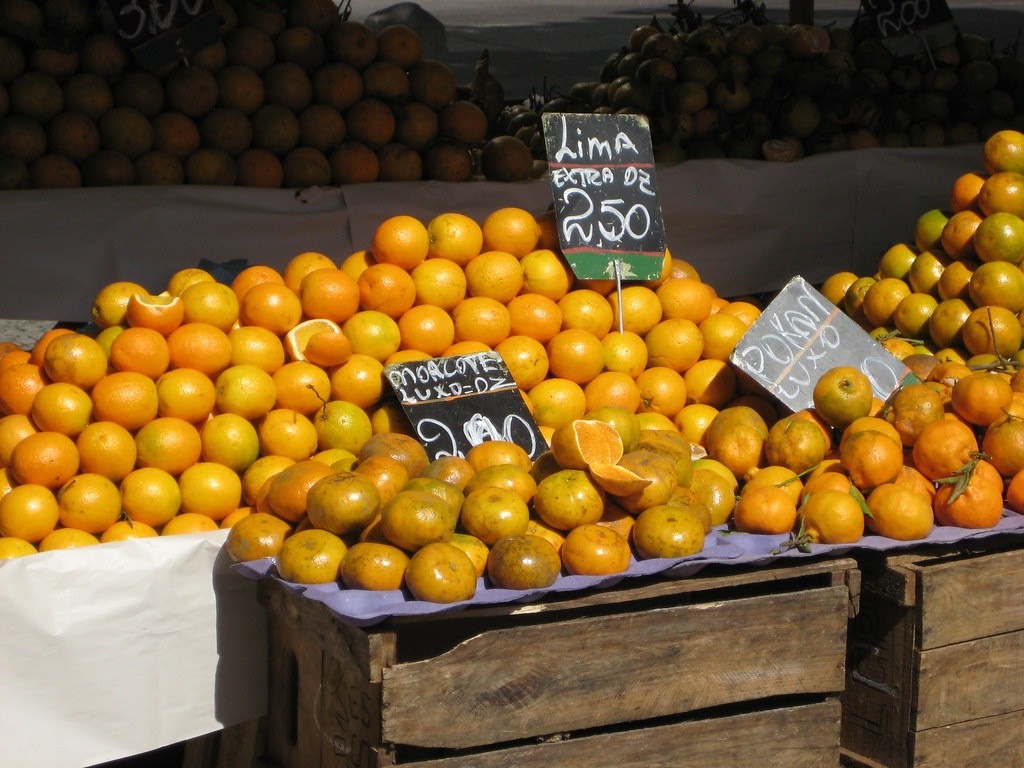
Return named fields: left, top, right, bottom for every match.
left=253, top=535, right=1023, bottom=766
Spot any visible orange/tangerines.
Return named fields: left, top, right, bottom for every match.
left=0, top=0, right=1024, bottom=188
left=0, top=129, right=1024, bottom=605
